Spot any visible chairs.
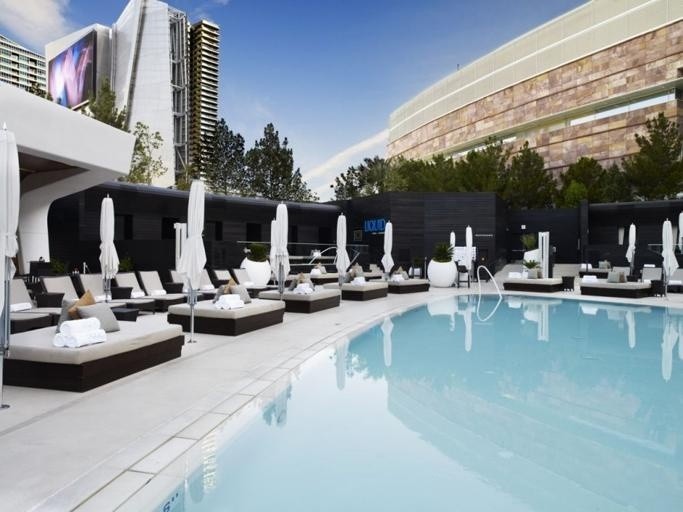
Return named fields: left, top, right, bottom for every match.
left=5, top=270, right=185, bottom=333
left=169, top=266, right=279, bottom=299
left=610, top=266, right=683, bottom=293
left=285, top=262, right=422, bottom=283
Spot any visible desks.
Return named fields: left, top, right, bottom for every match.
left=259, top=286, right=340, bottom=315
left=371, top=278, right=427, bottom=295
left=322, top=280, right=388, bottom=302
left=580, top=279, right=656, bottom=299
left=169, top=292, right=286, bottom=337
left=504, top=276, right=563, bottom=293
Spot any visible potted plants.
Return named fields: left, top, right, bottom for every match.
left=427, top=241, right=459, bottom=288
left=519, top=232, right=538, bottom=262
left=240, top=242, right=272, bottom=286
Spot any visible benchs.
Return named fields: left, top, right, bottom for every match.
left=3, top=320, right=183, bottom=393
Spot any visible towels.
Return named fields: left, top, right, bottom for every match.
left=54, top=318, right=106, bottom=347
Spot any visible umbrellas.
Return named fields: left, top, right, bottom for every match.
left=463, top=296, right=472, bottom=353
left=624, top=221, right=637, bottom=282
left=449, top=230, right=455, bottom=250
left=625, top=310, right=639, bottom=351
left=96, top=193, right=121, bottom=301
left=661, top=216, right=679, bottom=300
left=380, top=220, right=394, bottom=281
left=335, top=212, right=350, bottom=287
left=465, top=224, right=473, bottom=287
left=273, top=203, right=290, bottom=300
left=270, top=218, right=277, bottom=283
left=176, top=179, right=206, bottom=344
left=380, top=317, right=396, bottom=369
left=659, top=318, right=678, bottom=385
left=0, top=120, right=20, bottom=405
left=334, top=337, right=348, bottom=392
